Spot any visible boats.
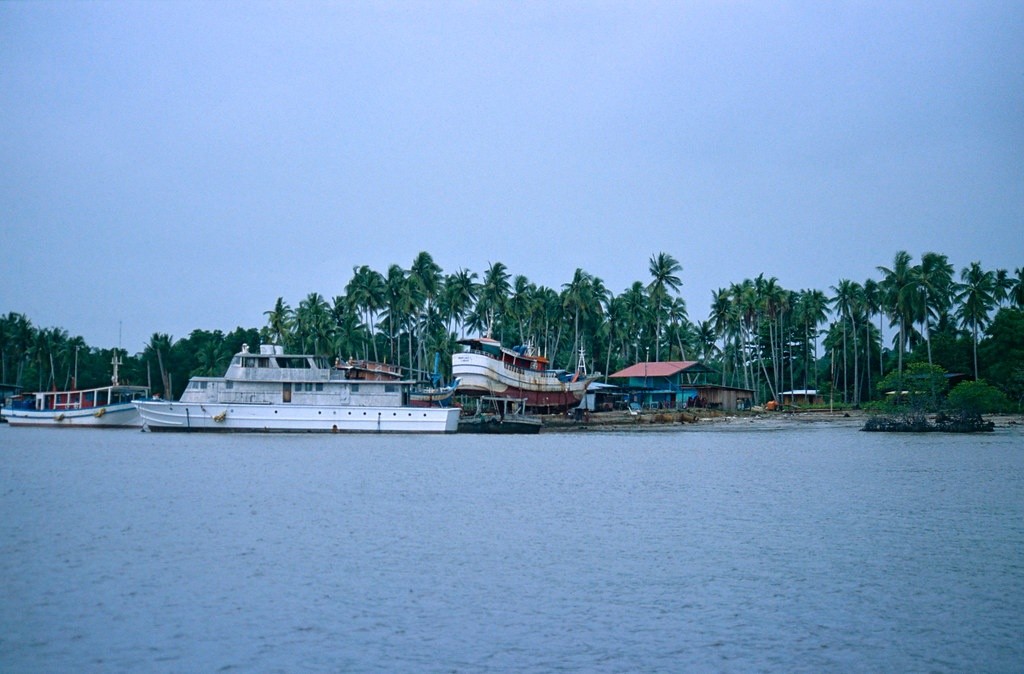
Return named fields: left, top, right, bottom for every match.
left=450, top=333, right=605, bottom=414
left=0, top=385, right=151, bottom=428
left=131, top=341, right=464, bottom=435
left=457, top=394, right=545, bottom=435
